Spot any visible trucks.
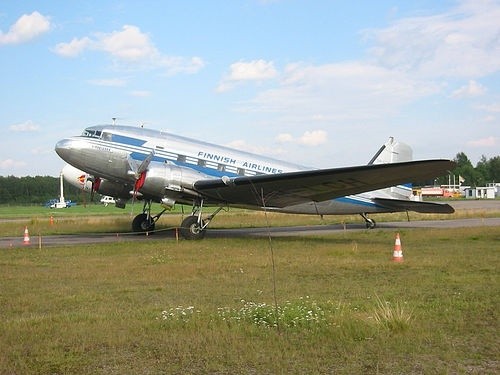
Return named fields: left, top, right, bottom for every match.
left=421, top=187, right=453, bottom=198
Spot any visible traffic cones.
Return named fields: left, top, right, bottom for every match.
left=392, top=231, right=404, bottom=262
left=23, top=224, right=32, bottom=245
left=50, top=216, right=54, bottom=226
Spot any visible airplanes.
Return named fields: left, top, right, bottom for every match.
left=55, top=117, right=455, bottom=239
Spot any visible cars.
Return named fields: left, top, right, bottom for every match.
left=50, top=203, right=71, bottom=209
left=100, top=196, right=119, bottom=204
left=452, top=192, right=464, bottom=198
